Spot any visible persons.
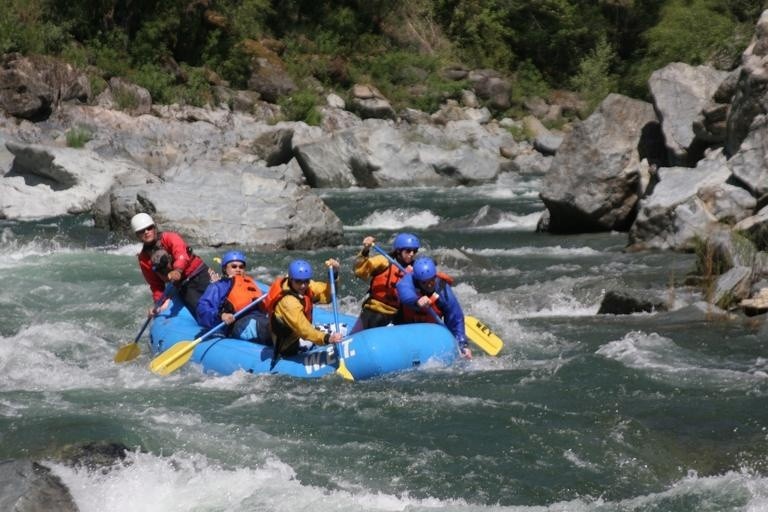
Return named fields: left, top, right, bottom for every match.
left=395, top=256, right=474, bottom=362
left=263, top=258, right=343, bottom=359
left=196, top=250, right=271, bottom=345
left=132, top=212, right=211, bottom=319
left=350, top=233, right=420, bottom=334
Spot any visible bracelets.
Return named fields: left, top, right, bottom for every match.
left=324, top=334, right=330, bottom=344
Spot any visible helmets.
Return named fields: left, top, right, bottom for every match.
left=392, top=232, right=419, bottom=254
left=288, top=260, right=313, bottom=281
left=130, top=212, right=154, bottom=233
left=413, top=256, right=436, bottom=281
left=222, top=250, right=246, bottom=270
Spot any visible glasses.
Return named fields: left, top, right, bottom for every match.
left=404, top=248, right=418, bottom=254
left=137, top=225, right=154, bottom=234
left=293, top=279, right=312, bottom=285
left=230, top=264, right=244, bottom=270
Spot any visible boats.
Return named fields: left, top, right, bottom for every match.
left=146, top=277, right=461, bottom=383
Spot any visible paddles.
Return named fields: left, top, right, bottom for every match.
left=371, top=242, right=503, bottom=357
left=326, top=262, right=354, bottom=382
left=149, top=292, right=267, bottom=376
left=115, top=279, right=173, bottom=363
